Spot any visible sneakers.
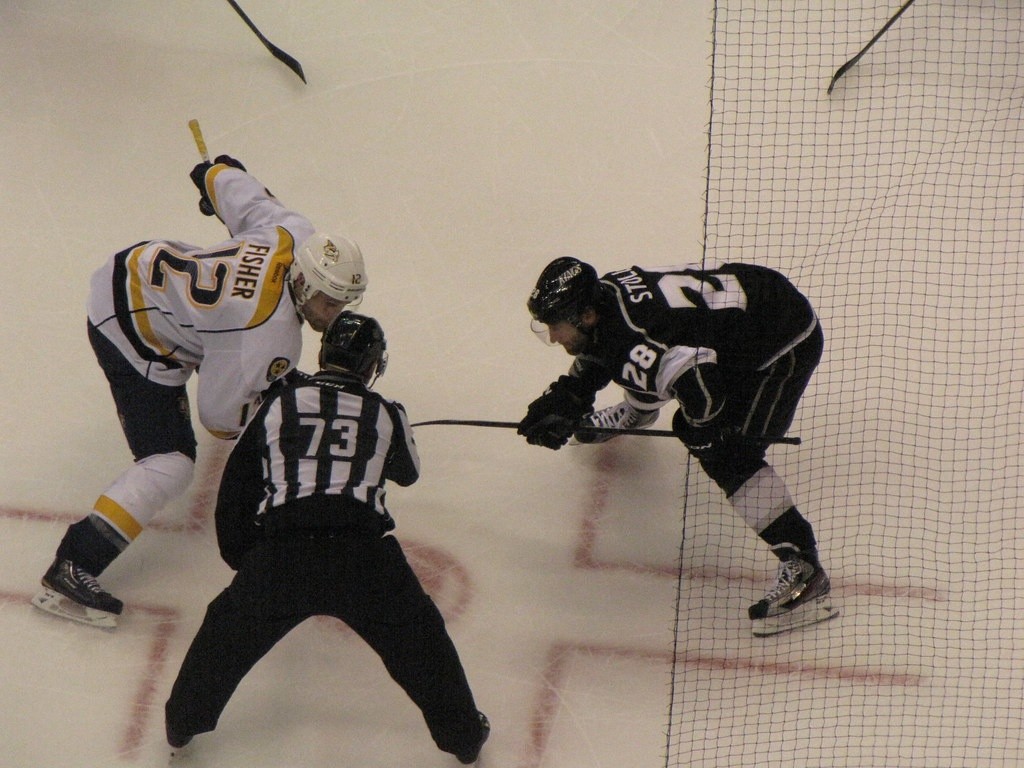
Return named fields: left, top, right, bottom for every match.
left=456, top=711, right=491, bottom=768
left=570, top=400, right=661, bottom=448
left=748, top=548, right=840, bottom=636
left=165, top=722, right=193, bottom=764
left=30, top=558, right=123, bottom=628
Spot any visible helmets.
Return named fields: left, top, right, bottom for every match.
left=528, top=255, right=599, bottom=323
left=295, top=230, right=366, bottom=303
left=317, top=308, right=387, bottom=380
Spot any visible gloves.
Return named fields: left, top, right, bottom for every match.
left=672, top=407, right=721, bottom=460
left=191, top=154, right=247, bottom=216
left=517, top=376, right=595, bottom=450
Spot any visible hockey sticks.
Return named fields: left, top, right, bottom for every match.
left=189, top=118, right=211, bottom=166
left=403, top=413, right=803, bottom=451
left=223, top=0, right=308, bottom=85
left=828, top=0, right=913, bottom=93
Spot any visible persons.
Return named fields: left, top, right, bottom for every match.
left=30, top=156, right=367, bottom=626
left=165, top=309, right=489, bottom=764
left=517, top=257, right=839, bottom=636
